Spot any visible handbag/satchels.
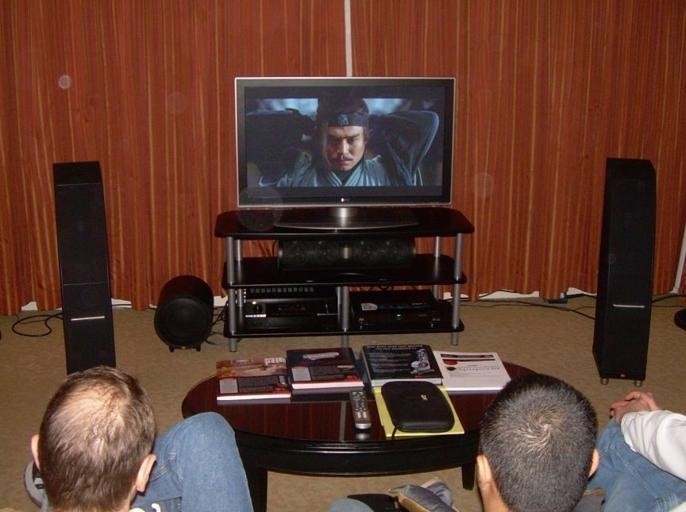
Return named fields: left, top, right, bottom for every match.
left=382, top=381, right=454, bottom=440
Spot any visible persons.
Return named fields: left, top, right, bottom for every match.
left=28, top=364, right=254, bottom=512
left=584, top=390, right=686, bottom=511
left=247, top=98, right=440, bottom=188
left=474, top=372, right=601, bottom=511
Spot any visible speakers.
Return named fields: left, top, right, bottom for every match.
left=52, top=160, right=116, bottom=376
left=154, top=274, right=213, bottom=353
left=591, top=158, right=657, bottom=387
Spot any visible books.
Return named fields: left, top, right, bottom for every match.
left=361, top=344, right=443, bottom=387
left=432, top=350, right=512, bottom=392
left=216, top=357, right=293, bottom=400
left=286, top=347, right=366, bottom=391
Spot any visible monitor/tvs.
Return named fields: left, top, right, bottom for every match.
left=234, top=77, right=456, bottom=230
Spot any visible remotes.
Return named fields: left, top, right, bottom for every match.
left=349, top=390, right=372, bottom=430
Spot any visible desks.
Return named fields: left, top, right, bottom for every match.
left=213, top=207, right=475, bottom=352
